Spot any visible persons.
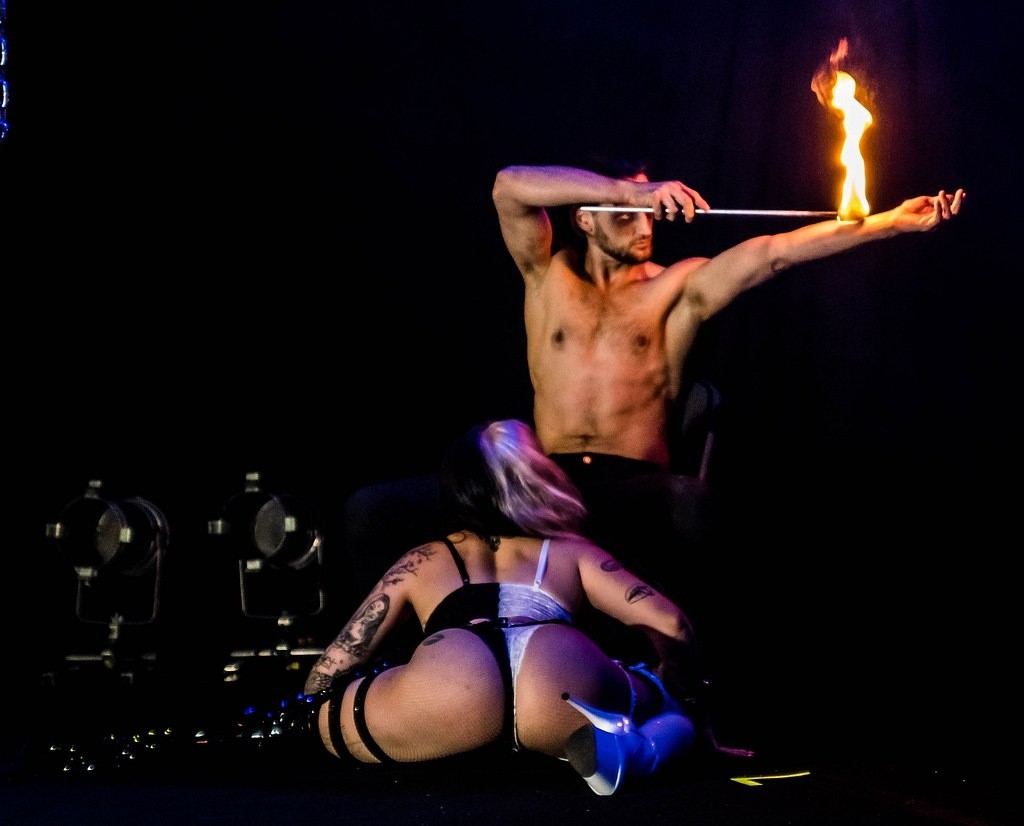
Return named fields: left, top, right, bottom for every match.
left=296, top=417, right=725, bottom=826
left=496, top=136, right=969, bottom=784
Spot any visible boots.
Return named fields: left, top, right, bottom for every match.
left=558, top=662, right=693, bottom=796
left=46, top=689, right=326, bottom=785
